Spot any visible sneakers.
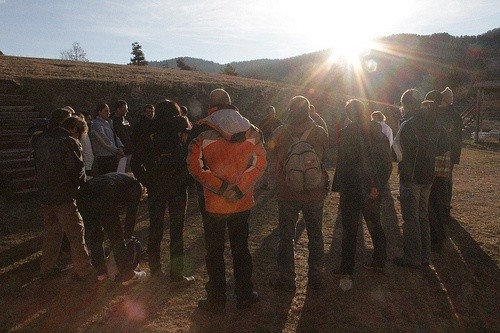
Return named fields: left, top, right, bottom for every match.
left=97, top=273, right=115, bottom=283
left=122, top=270, right=146, bottom=285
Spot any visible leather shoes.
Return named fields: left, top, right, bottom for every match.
left=150, top=270, right=166, bottom=282
left=172, top=276, right=196, bottom=290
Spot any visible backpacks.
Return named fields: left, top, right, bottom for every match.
left=106, top=236, right=142, bottom=284
left=274, top=124, right=330, bottom=202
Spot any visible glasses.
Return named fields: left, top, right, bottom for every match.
left=145, top=110, right=155, bottom=113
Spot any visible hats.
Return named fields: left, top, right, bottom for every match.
left=421, top=89, right=442, bottom=105
left=209, top=89, right=233, bottom=107
left=441, top=87, right=453, bottom=105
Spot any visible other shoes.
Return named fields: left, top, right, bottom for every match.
left=198, top=299, right=225, bottom=315
left=392, top=256, right=420, bottom=269
left=332, top=269, right=353, bottom=278
left=362, top=261, right=386, bottom=272
left=270, top=278, right=296, bottom=292
left=237, top=291, right=259, bottom=309
left=40, top=265, right=58, bottom=279
left=80, top=268, right=97, bottom=281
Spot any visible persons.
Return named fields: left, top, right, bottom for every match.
left=184, top=89, right=267, bottom=313
left=25, top=86, right=462, bottom=291
